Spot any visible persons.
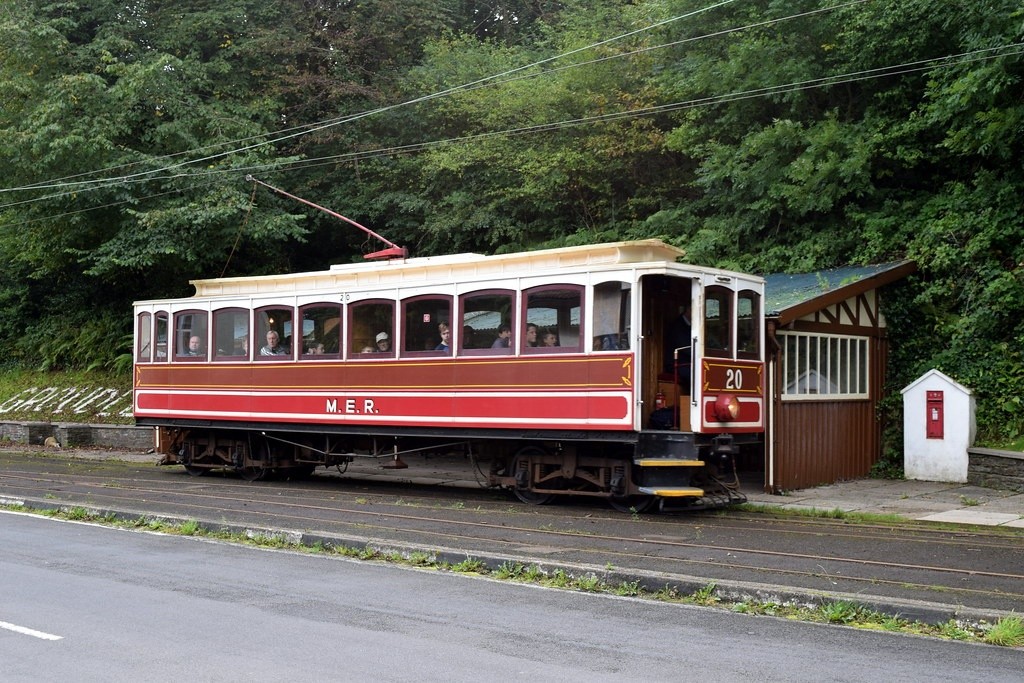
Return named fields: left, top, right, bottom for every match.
left=304, top=342, right=326, bottom=356
left=435, top=320, right=451, bottom=350
left=540, top=327, right=558, bottom=347
left=490, top=323, right=512, bottom=349
left=376, top=332, right=392, bottom=352
left=185, top=334, right=208, bottom=358
left=259, top=330, right=293, bottom=356
left=524, top=322, right=538, bottom=347
left=462, top=324, right=479, bottom=349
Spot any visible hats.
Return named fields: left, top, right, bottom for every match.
left=376, top=332, right=388, bottom=343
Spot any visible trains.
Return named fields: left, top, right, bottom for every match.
left=132, top=238, right=768, bottom=517
